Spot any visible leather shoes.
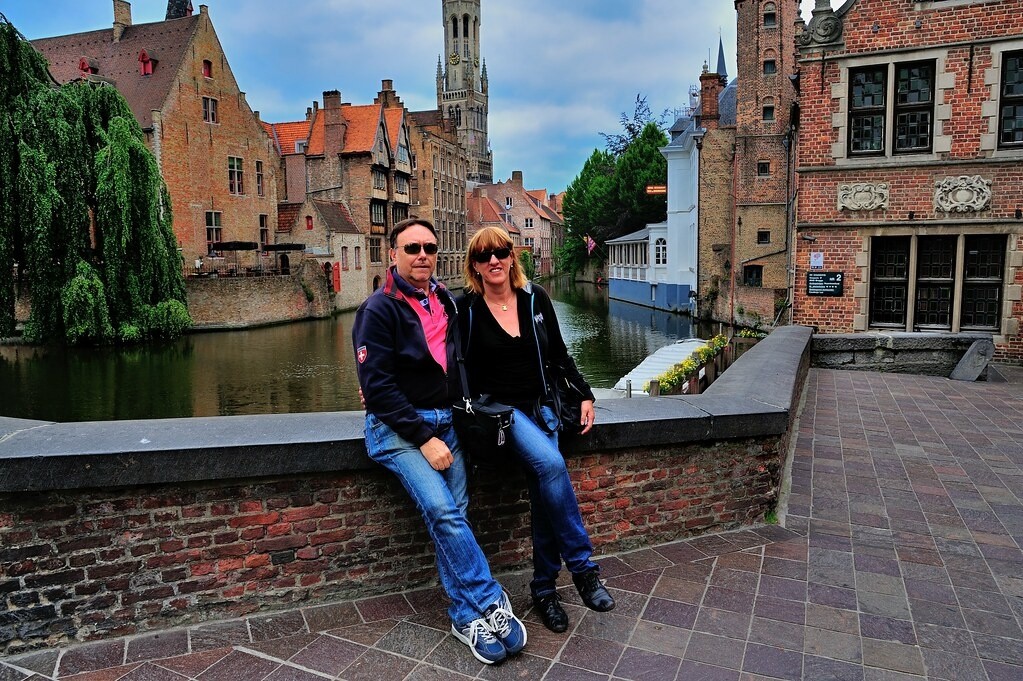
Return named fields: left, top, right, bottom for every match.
left=572, top=570, right=615, bottom=612
left=533, top=591, right=568, bottom=633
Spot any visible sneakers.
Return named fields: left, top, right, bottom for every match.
left=483, top=589, right=527, bottom=654
left=451, top=615, right=506, bottom=664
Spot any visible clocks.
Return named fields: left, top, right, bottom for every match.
left=449, top=53, right=460, bottom=65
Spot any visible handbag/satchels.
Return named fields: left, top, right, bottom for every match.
left=454, top=393, right=513, bottom=451
left=533, top=364, right=592, bottom=440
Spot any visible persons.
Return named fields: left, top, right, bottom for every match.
left=358, top=227, right=615, bottom=632
left=351, top=218, right=529, bottom=665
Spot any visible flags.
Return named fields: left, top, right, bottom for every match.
left=583, top=237, right=587, bottom=242
left=588, top=235, right=595, bottom=254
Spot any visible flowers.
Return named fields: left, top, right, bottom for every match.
left=642, top=334, right=727, bottom=394
left=740, top=329, right=767, bottom=339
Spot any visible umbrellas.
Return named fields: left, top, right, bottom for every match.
left=213, top=241, right=259, bottom=278
left=262, top=242, right=306, bottom=260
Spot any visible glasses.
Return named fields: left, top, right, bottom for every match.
left=394, top=243, right=438, bottom=254
left=474, top=247, right=511, bottom=263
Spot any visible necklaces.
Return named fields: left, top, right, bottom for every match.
left=487, top=295, right=513, bottom=311
